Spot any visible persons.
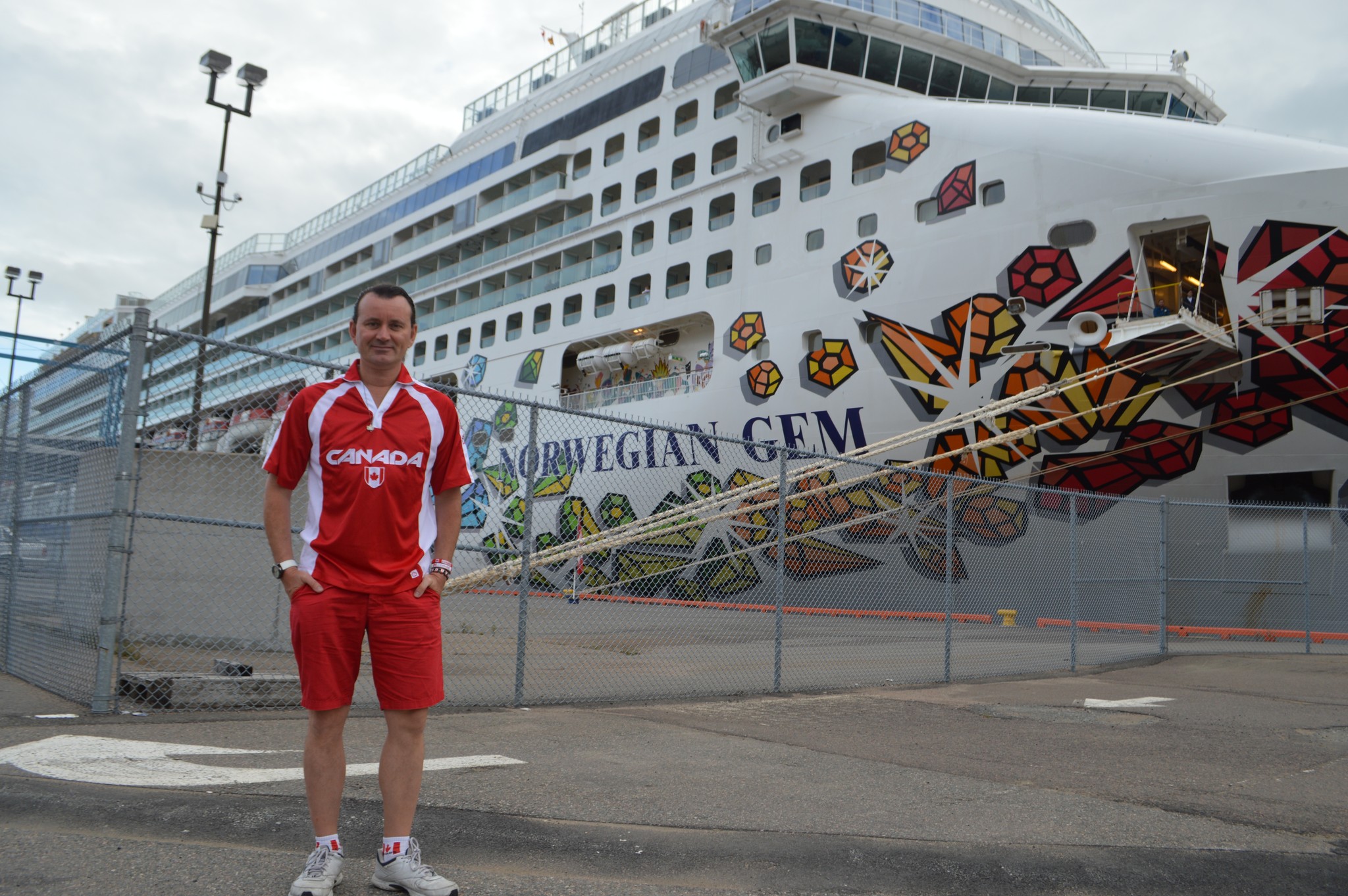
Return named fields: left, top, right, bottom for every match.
left=260, top=285, right=462, bottom=896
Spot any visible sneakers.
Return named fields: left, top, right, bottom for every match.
left=288, top=845, right=344, bottom=896
left=372, top=837, right=459, bottom=896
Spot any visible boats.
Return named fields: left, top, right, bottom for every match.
left=147, top=390, right=299, bottom=453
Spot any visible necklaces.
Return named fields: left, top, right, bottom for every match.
left=363, top=383, right=378, bottom=432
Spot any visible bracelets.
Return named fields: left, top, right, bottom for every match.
left=429, top=558, right=452, bottom=581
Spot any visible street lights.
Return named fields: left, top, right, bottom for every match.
left=184, top=49, right=267, bottom=450
left=0, top=265, right=45, bottom=461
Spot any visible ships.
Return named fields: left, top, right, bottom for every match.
left=0, top=0, right=1348, bottom=644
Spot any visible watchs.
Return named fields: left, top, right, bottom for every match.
left=271, top=560, right=297, bottom=580
